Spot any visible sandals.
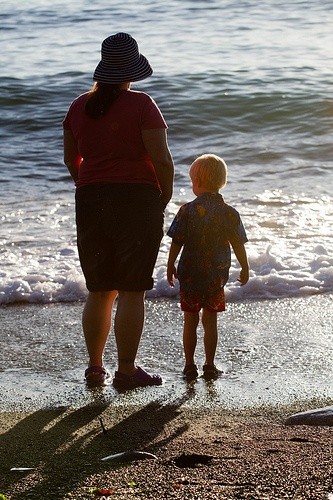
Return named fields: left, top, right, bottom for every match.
left=112, top=367, right=162, bottom=388
left=182, top=365, right=198, bottom=381
left=85, top=366, right=106, bottom=386
left=203, top=365, right=224, bottom=380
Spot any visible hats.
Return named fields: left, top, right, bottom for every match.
left=93, top=32, right=153, bottom=84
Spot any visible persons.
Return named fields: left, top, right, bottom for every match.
left=167, top=154, right=250, bottom=379
left=62, top=33, right=174, bottom=388
